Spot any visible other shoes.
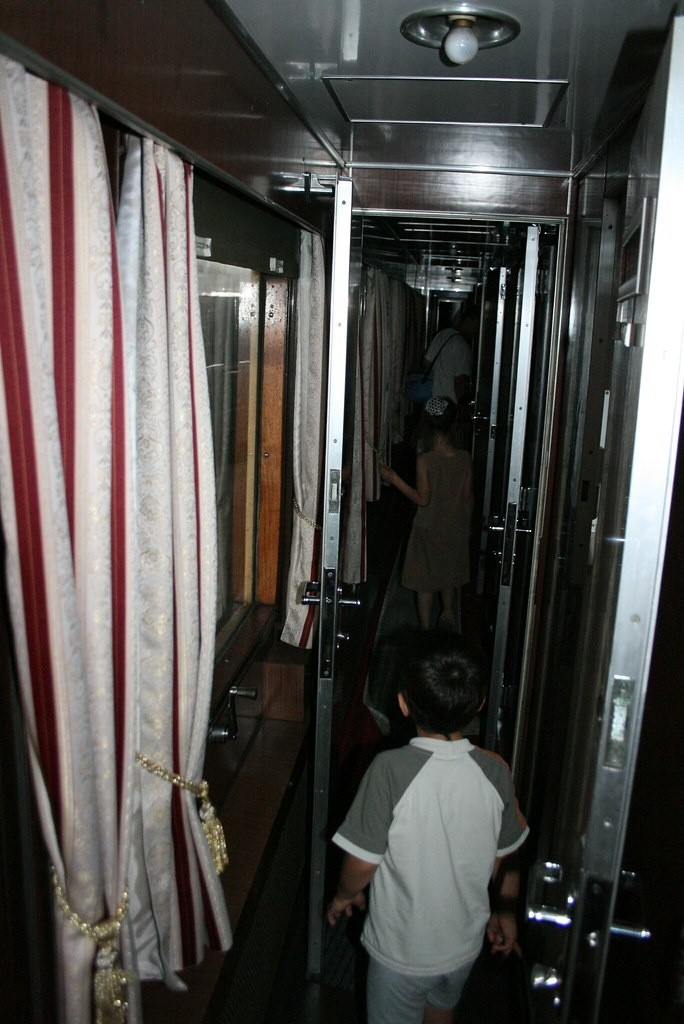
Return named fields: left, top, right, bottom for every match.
left=437, top=609, right=458, bottom=630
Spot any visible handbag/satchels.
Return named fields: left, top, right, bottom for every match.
left=405, top=372, right=432, bottom=403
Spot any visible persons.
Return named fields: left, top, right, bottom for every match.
left=323, top=626, right=529, bottom=1023
left=378, top=310, right=476, bottom=629
